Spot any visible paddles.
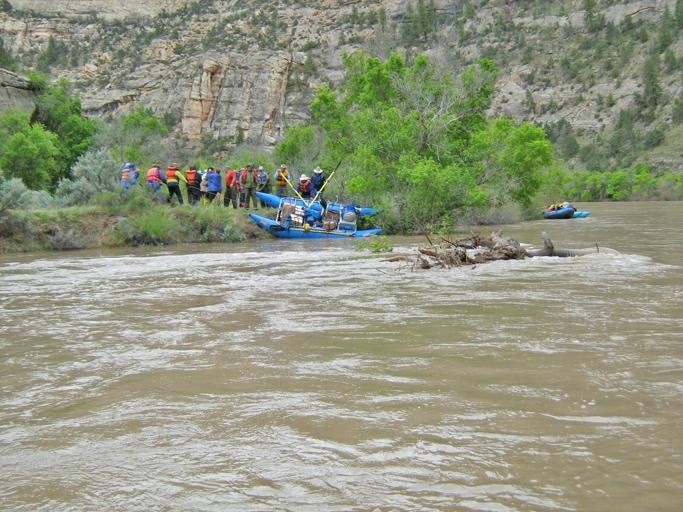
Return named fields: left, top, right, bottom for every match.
left=268, top=224, right=354, bottom=237
left=308, top=160, right=341, bottom=208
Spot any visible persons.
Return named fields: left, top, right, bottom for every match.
left=165, top=164, right=188, bottom=204
left=549, top=203, right=563, bottom=210
left=274, top=163, right=293, bottom=196
left=121, top=163, right=140, bottom=191
left=239, top=165, right=259, bottom=210
left=256, top=165, right=272, bottom=208
left=186, top=164, right=222, bottom=205
left=146, top=164, right=167, bottom=204
left=236, top=168, right=246, bottom=207
left=294, top=173, right=314, bottom=198
left=224, top=166, right=238, bottom=208
left=311, top=167, right=326, bottom=198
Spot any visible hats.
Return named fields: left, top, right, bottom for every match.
left=281, top=164, right=287, bottom=168
left=313, top=167, right=323, bottom=174
left=300, top=175, right=308, bottom=180
left=259, top=166, right=264, bottom=171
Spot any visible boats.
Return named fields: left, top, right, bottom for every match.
left=246, top=190, right=385, bottom=240
left=542, top=207, right=592, bottom=219
